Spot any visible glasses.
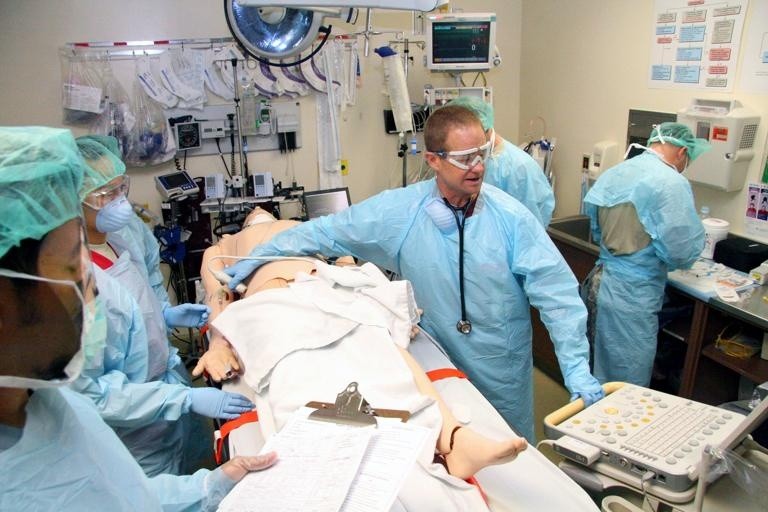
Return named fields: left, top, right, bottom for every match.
left=436, top=142, right=492, bottom=169
left=80, top=176, right=129, bottom=212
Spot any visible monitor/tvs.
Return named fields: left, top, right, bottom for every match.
left=301, top=187, right=352, bottom=221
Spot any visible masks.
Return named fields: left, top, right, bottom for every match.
left=426, top=198, right=461, bottom=234
left=96, top=193, right=133, bottom=233
left=0, top=269, right=104, bottom=390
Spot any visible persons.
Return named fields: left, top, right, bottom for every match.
left=579, top=121, right=705, bottom=392
left=1, top=126, right=278, bottom=512
left=442, top=95, right=557, bottom=232
left=219, top=106, right=604, bottom=446
left=75, top=134, right=169, bottom=302
left=746, top=195, right=768, bottom=220
left=191, top=206, right=529, bottom=480
left=69, top=135, right=256, bottom=479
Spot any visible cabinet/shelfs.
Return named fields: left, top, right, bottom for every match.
left=686, top=301, right=768, bottom=406
left=648, top=280, right=703, bottom=396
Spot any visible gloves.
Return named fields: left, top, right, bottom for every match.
left=221, top=258, right=262, bottom=289
left=570, top=376, right=605, bottom=408
left=189, top=387, right=256, bottom=420
left=163, top=303, right=212, bottom=328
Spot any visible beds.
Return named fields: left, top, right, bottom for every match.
left=194, top=276, right=601, bottom=512
